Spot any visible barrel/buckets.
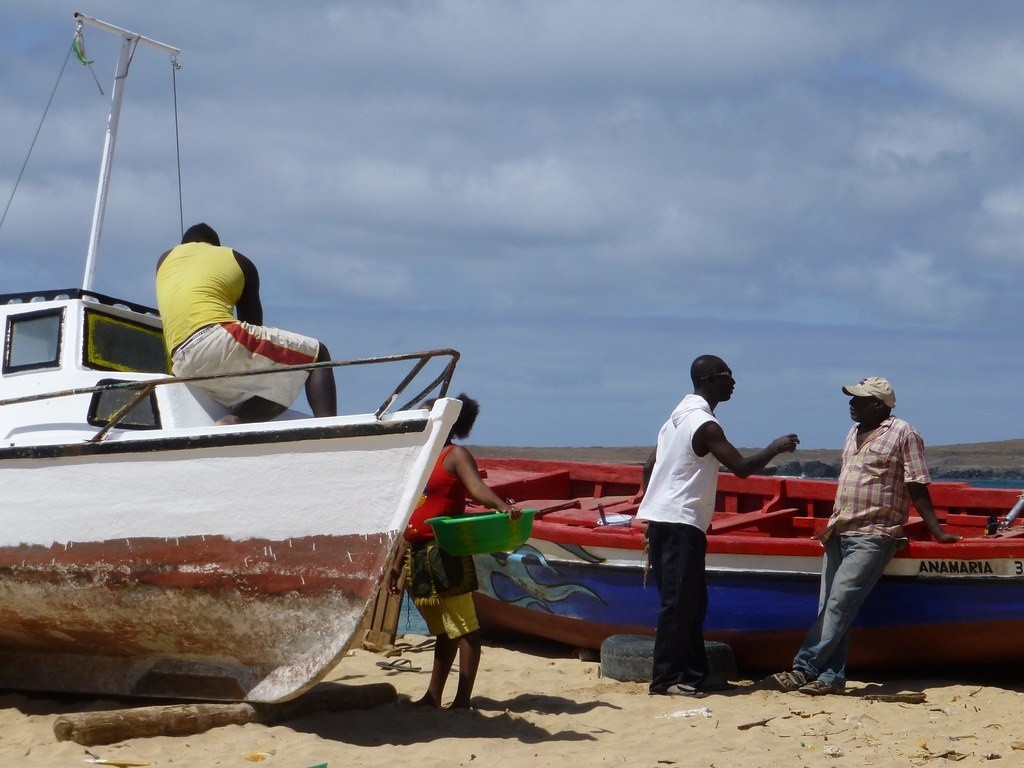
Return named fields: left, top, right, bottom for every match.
left=596, top=512, right=632, bottom=529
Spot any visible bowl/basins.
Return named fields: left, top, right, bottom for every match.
left=424, top=509, right=542, bottom=555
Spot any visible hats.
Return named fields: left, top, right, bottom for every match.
left=842, top=376, right=895, bottom=409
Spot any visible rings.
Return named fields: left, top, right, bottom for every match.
left=512, top=508, right=515, bottom=511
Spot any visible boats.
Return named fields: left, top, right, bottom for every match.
left=1, top=12, right=464, bottom=705
left=415, top=452, right=1024, bottom=677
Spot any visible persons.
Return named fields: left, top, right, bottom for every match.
left=771, top=377, right=964, bottom=696
left=154, top=221, right=338, bottom=425
left=387, top=391, right=524, bottom=709
left=635, top=354, right=799, bottom=696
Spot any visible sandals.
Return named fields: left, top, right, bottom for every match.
left=773, top=670, right=809, bottom=691
left=799, top=680, right=833, bottom=695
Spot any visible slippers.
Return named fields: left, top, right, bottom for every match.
left=693, top=674, right=737, bottom=690
left=650, top=684, right=704, bottom=698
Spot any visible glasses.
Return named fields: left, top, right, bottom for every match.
left=700, top=369, right=732, bottom=379
